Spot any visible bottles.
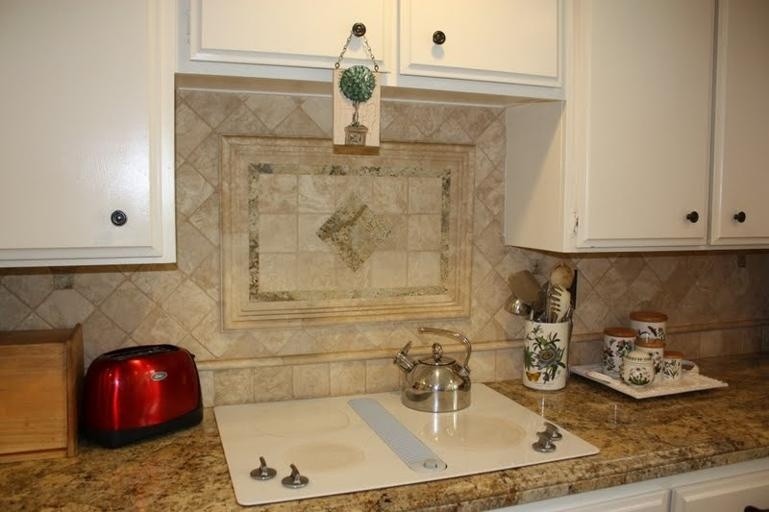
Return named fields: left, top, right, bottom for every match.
left=604, top=307, right=685, bottom=388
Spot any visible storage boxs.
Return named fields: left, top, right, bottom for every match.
left=0, top=323, right=84, bottom=463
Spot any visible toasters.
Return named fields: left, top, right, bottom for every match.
left=82, top=342, right=201, bottom=450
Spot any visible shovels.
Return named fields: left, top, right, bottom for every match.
left=508, top=270, right=546, bottom=313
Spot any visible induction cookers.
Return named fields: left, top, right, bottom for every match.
left=212, top=381, right=601, bottom=507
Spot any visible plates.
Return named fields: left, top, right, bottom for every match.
left=570, top=357, right=728, bottom=402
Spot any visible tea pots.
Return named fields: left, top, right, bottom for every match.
left=393, top=324, right=473, bottom=414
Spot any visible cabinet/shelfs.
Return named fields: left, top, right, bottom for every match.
left=0, top=0, right=177, bottom=268
left=174, top=0, right=566, bottom=106
left=504, top=0, right=769, bottom=254
left=552, top=469, right=769, bottom=512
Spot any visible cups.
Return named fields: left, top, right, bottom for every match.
left=521, top=319, right=570, bottom=392
